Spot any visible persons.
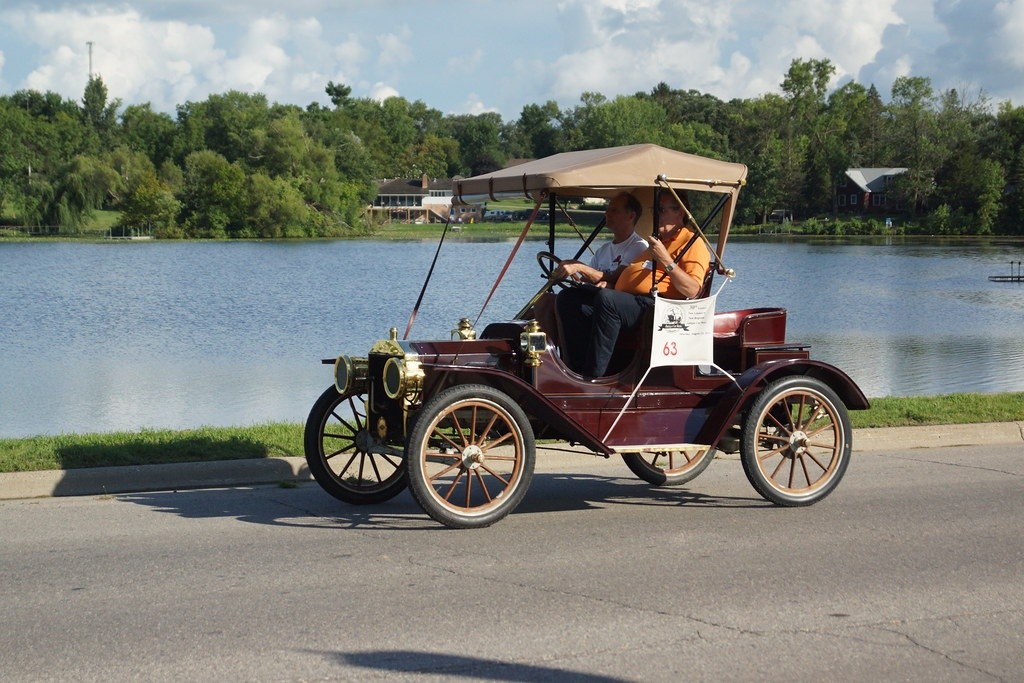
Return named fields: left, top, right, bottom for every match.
left=533, top=192, right=650, bottom=372
left=556, top=185, right=708, bottom=381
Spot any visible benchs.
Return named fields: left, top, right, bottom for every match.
left=615, top=262, right=717, bottom=349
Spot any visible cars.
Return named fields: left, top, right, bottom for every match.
left=302, top=143, right=871, bottom=530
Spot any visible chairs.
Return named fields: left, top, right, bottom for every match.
left=710, top=306, right=786, bottom=349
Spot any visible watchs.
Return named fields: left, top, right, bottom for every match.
left=666, top=262, right=677, bottom=272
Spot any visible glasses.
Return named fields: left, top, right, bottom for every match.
left=649, top=206, right=677, bottom=214
